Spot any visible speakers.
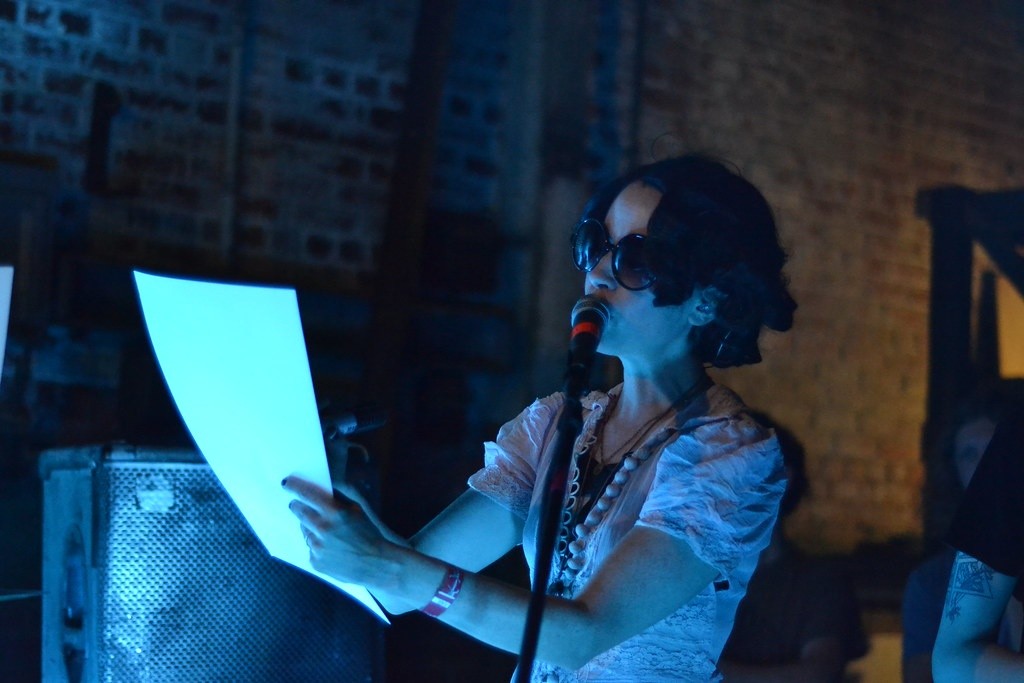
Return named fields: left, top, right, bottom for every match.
left=36, top=442, right=385, bottom=683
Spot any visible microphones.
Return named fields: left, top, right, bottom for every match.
left=564, top=293, right=610, bottom=372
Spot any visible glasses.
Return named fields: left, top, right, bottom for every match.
left=570, top=218, right=662, bottom=292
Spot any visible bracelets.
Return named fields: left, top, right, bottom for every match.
left=422, top=564, right=463, bottom=617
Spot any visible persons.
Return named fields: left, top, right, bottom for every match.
left=280, top=152, right=798, bottom=683
left=715, top=384, right=1024, bottom=683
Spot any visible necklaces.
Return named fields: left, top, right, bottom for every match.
left=549, top=374, right=709, bottom=595
